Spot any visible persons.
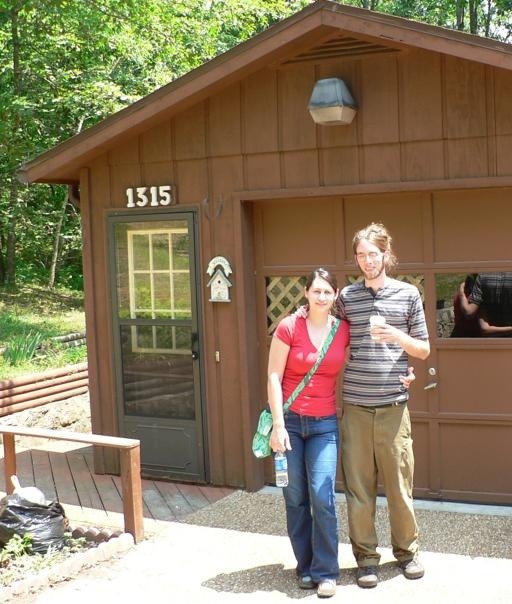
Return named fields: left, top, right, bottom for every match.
left=266, top=267, right=417, bottom=598
left=294, top=221, right=431, bottom=588
left=459, top=272, right=512, bottom=338
left=449, top=272, right=512, bottom=338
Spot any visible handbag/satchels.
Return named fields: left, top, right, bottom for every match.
left=252, top=410, right=273, bottom=459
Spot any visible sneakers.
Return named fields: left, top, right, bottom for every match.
left=297, top=575, right=315, bottom=589
left=357, top=566, right=378, bottom=587
left=317, top=578, right=336, bottom=597
left=402, top=559, right=423, bottom=579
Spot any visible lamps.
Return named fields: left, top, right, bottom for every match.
left=307, top=80, right=357, bottom=125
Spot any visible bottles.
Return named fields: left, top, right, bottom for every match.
left=274, top=450, right=291, bottom=489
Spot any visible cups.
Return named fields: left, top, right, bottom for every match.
left=370, top=314, right=386, bottom=340
